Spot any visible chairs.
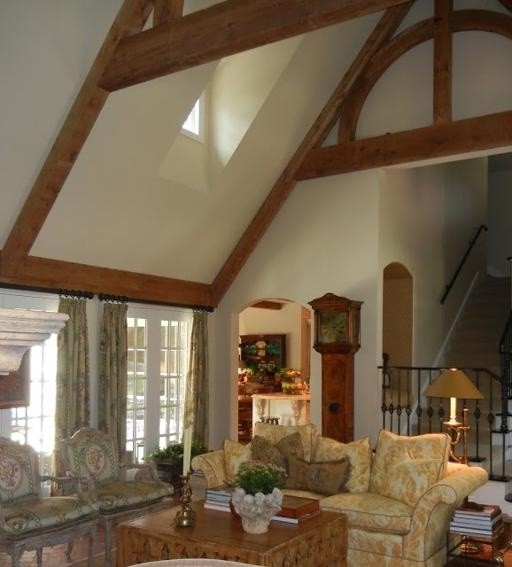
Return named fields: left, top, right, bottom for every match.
left=0, top=435, right=100, bottom=567
left=51, top=424, right=175, bottom=567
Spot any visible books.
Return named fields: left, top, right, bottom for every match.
left=203, top=482, right=324, bottom=527
left=448, top=502, right=508, bottom=544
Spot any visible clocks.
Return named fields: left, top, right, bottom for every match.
left=306, top=292, right=364, bottom=443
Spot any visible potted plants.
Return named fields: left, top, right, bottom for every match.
left=140, top=437, right=208, bottom=483
left=230, top=460, right=289, bottom=535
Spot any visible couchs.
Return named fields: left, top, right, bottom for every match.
left=190, top=450, right=489, bottom=567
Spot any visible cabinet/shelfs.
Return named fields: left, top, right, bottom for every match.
left=238, top=333, right=310, bottom=445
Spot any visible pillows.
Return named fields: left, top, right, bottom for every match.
left=223, top=421, right=452, bottom=508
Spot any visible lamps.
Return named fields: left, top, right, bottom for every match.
left=422, top=366, right=486, bottom=504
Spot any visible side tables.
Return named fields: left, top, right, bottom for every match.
left=446, top=519, right=512, bottom=566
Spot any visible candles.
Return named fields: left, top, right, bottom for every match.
left=182, top=427, right=191, bottom=475
